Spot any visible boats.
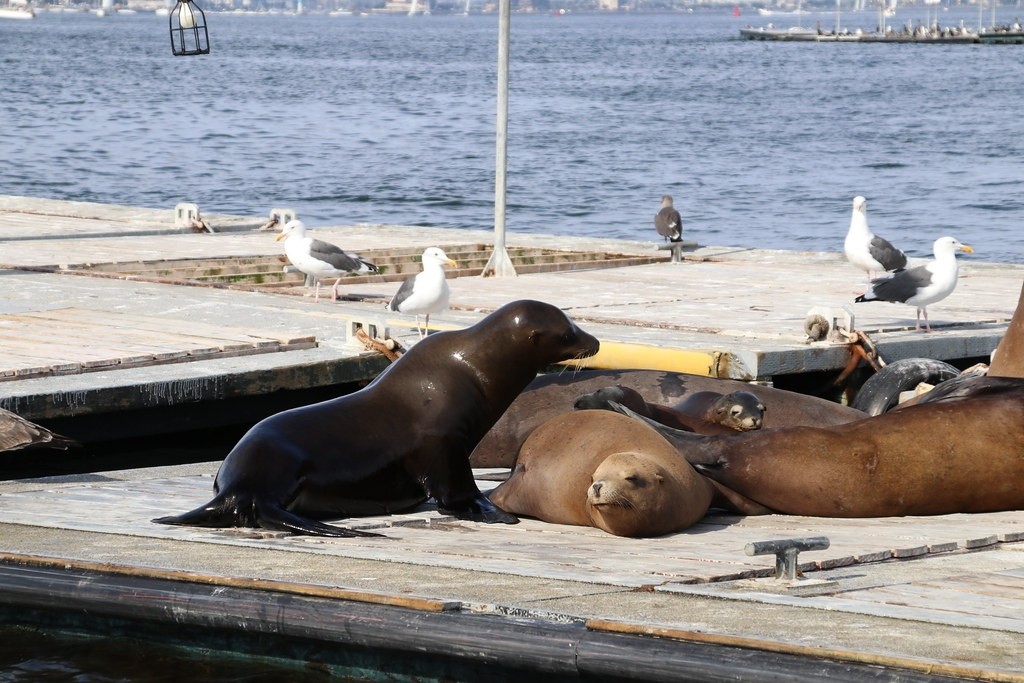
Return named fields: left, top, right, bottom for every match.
left=1, top=6, right=38, bottom=21
left=737, top=26, right=1024, bottom=45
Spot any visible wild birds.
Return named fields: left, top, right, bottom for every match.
left=843, top=196, right=974, bottom=334
left=0, top=407, right=83, bottom=453
left=654, top=195, right=684, bottom=242
left=277, top=220, right=380, bottom=301
left=385, top=247, right=457, bottom=341
